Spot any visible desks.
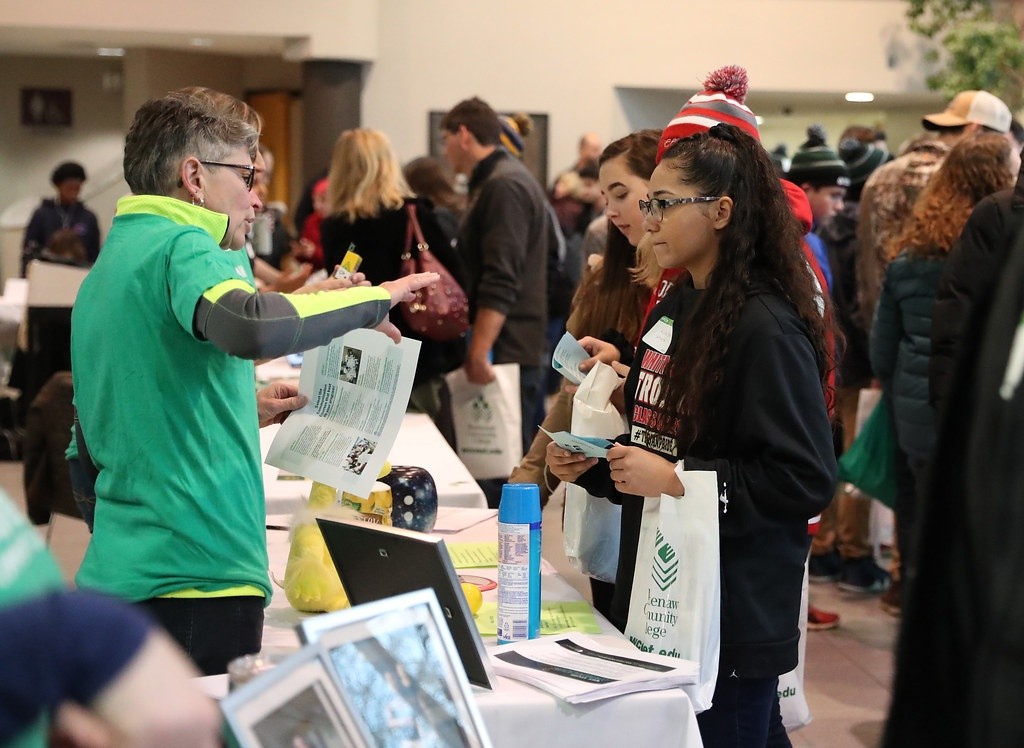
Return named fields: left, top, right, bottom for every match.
left=254, top=354, right=704, bottom=748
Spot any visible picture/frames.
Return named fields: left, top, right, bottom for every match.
left=218, top=588, right=495, bottom=747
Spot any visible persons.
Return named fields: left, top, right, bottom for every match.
left=21, top=161, right=102, bottom=278
left=546, top=124, right=838, bottom=748
left=247, top=96, right=564, bottom=510
left=509, top=65, right=842, bottom=509
left=0, top=491, right=222, bottom=748
left=789, top=89, right=1024, bottom=748
left=70, top=87, right=439, bottom=674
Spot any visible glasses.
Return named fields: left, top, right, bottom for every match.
left=178, top=154, right=255, bottom=197
left=638, top=196, right=722, bottom=222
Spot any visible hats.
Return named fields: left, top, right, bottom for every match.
left=922, top=91, right=1011, bottom=133
left=788, top=125, right=850, bottom=187
left=839, top=139, right=897, bottom=199
left=655, top=66, right=758, bottom=162
left=496, top=112, right=532, bottom=158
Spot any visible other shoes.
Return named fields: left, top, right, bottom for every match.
left=879, top=584, right=907, bottom=615
left=808, top=552, right=845, bottom=582
left=806, top=606, right=841, bottom=628
left=838, top=556, right=890, bottom=595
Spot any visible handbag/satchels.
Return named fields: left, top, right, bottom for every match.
left=773, top=561, right=813, bottom=730
left=620, top=460, right=721, bottom=716
left=404, top=203, right=470, bottom=337
left=560, top=363, right=632, bottom=584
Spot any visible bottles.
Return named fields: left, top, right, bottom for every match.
left=497, top=484, right=542, bottom=645
